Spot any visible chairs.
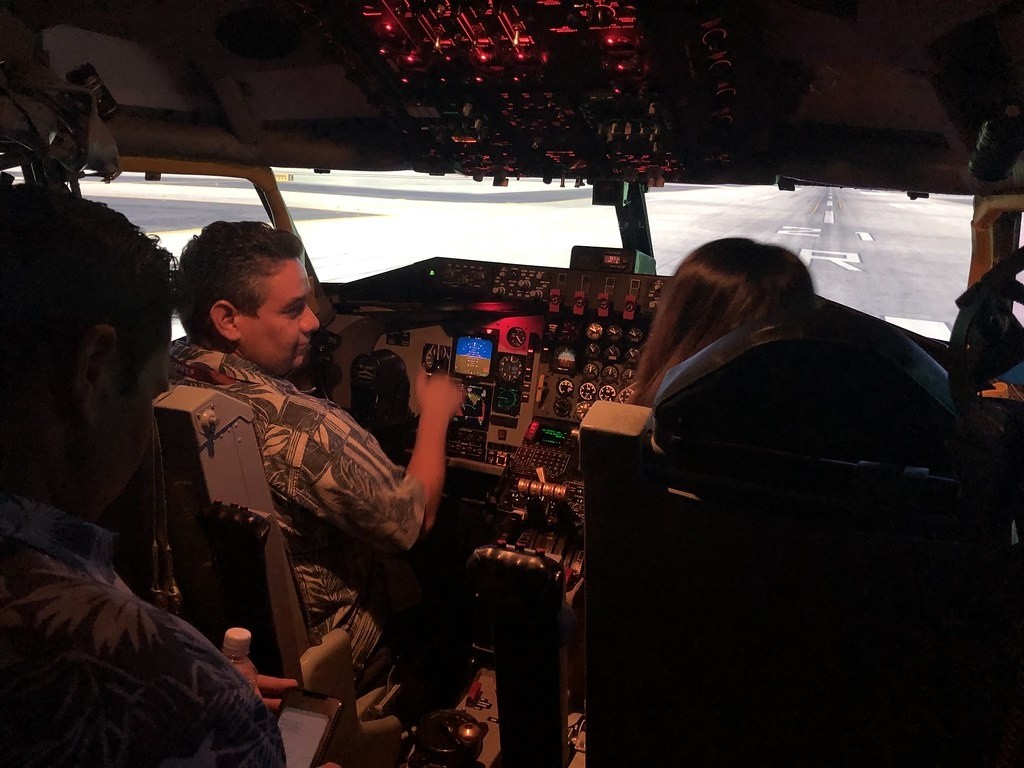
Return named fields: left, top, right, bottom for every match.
left=150, top=385, right=430, bottom=768
left=580, top=311, right=1024, bottom=768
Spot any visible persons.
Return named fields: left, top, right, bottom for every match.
left=631, top=238, right=809, bottom=410
left=160, top=221, right=462, bottom=667
left=0, top=181, right=299, bottom=768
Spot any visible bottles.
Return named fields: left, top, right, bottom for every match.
left=220, top=628, right=257, bottom=695
